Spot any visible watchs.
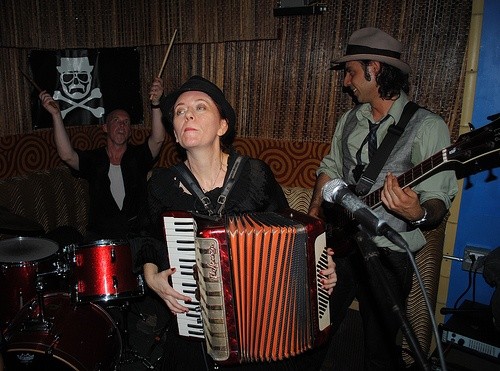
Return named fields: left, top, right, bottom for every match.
left=409, top=206, right=427, bottom=225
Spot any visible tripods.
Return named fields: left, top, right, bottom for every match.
left=104, top=301, right=156, bottom=371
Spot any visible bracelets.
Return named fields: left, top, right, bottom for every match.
left=150, top=101, right=161, bottom=109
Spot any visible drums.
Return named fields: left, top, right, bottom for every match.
left=0, top=290, right=125, bottom=371
left=71, top=234, right=147, bottom=306
left=0, top=235, right=61, bottom=311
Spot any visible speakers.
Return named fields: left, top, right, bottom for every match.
left=442, top=342, right=500, bottom=371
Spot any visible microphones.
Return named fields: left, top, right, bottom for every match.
left=322, top=177, right=411, bottom=247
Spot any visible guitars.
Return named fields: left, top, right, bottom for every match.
left=317, top=113, right=500, bottom=259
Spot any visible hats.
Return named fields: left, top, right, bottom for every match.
left=159, top=75, right=236, bottom=144
left=330, top=27, right=411, bottom=76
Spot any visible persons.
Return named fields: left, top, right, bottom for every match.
left=37, top=76, right=166, bottom=336
left=310, top=28, right=459, bottom=371
left=142, top=76, right=338, bottom=371
